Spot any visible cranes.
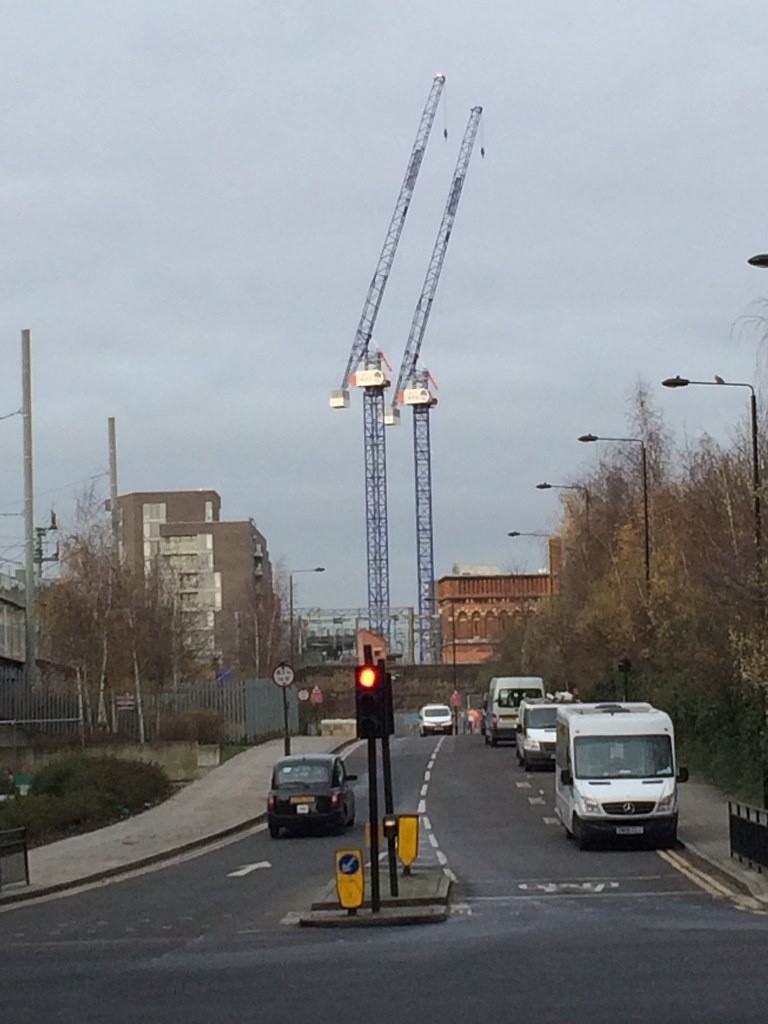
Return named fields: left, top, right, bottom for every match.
left=327, top=72, right=483, bottom=666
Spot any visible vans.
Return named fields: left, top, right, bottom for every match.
left=478, top=675, right=547, bottom=747
left=514, top=693, right=585, bottom=772
left=555, top=701, right=690, bottom=852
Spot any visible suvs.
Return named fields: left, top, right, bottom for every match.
left=264, top=752, right=358, bottom=837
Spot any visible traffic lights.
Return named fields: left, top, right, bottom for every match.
left=353, top=664, right=384, bottom=741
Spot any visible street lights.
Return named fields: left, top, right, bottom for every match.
left=578, top=433, right=651, bottom=603
left=289, top=567, right=324, bottom=669
left=508, top=531, right=554, bottom=617
left=663, top=375, right=766, bottom=655
left=537, top=481, right=591, bottom=557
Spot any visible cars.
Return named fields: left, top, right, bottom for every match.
left=418, top=702, right=453, bottom=737
